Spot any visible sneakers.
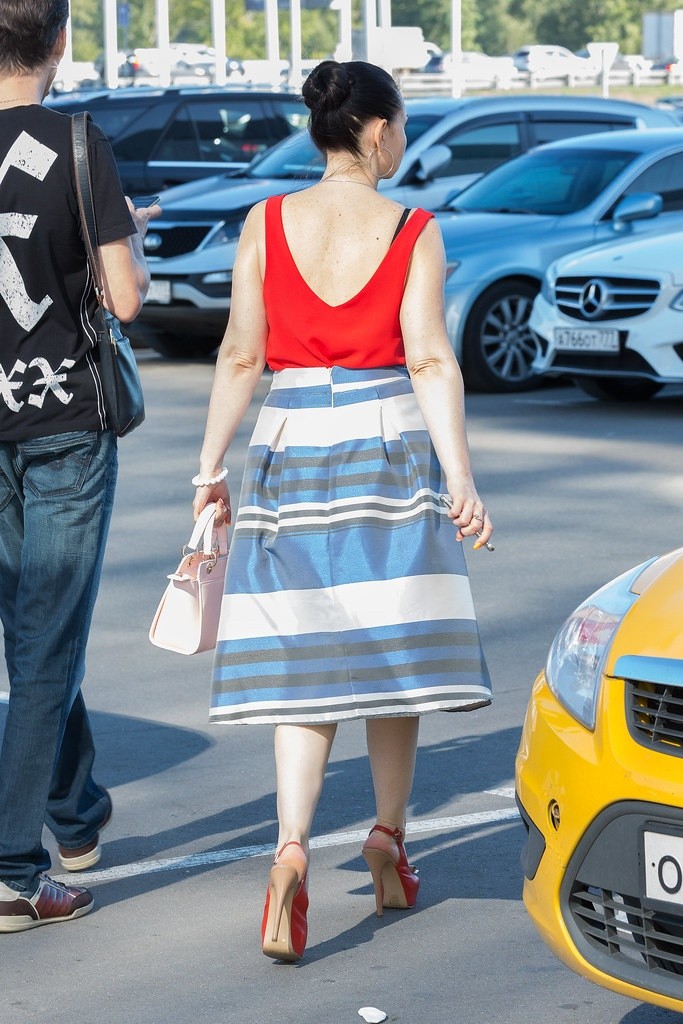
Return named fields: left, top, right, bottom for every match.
left=58, top=785, right=112, bottom=871
left=0, top=872, right=95, bottom=933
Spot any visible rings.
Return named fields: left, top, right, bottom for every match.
left=472, top=514, right=483, bottom=522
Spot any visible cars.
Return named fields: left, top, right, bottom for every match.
left=120, top=94, right=683, bottom=358
left=61, top=44, right=318, bottom=91
left=516, top=46, right=588, bottom=74
left=428, top=127, right=683, bottom=395
left=512, top=547, right=683, bottom=1017
left=425, top=52, right=516, bottom=84
left=526, top=229, right=682, bottom=401
left=44, top=87, right=314, bottom=201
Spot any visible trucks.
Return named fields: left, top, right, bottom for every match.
left=350, top=26, right=443, bottom=78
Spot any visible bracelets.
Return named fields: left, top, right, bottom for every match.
left=191, top=467, right=229, bottom=486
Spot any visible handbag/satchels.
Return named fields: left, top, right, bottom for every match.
left=147, top=501, right=229, bottom=656
left=72, top=110, right=146, bottom=438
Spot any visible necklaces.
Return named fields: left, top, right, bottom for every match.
left=0, top=97, right=34, bottom=102
left=322, top=179, right=375, bottom=190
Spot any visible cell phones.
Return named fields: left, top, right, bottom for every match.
left=131, top=195, right=161, bottom=210
left=440, top=494, right=496, bottom=552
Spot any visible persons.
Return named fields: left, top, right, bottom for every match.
left=0, top=0, right=161, bottom=932
left=193, top=62, right=492, bottom=960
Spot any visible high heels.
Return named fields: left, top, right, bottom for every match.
left=261, top=841, right=309, bottom=962
left=361, top=824, right=420, bottom=917
